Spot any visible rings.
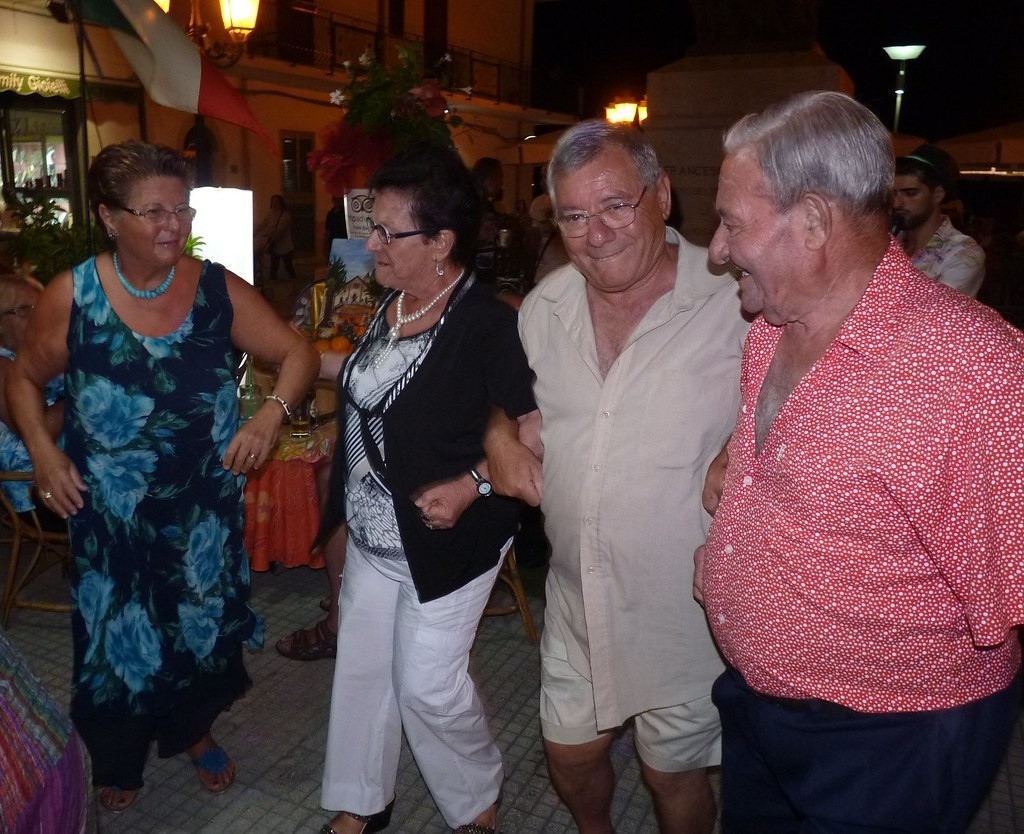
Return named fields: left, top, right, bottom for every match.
left=248, top=452, right=258, bottom=459
left=426, top=524, right=432, bottom=529
left=44, top=493, right=51, bottom=500
left=420, top=506, right=433, bottom=521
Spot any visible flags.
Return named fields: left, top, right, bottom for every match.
left=67, top=0, right=283, bottom=166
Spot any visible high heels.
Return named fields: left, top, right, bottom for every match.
left=453, top=791, right=501, bottom=834
left=319, top=791, right=396, bottom=834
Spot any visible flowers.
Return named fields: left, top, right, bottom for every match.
left=307, top=35, right=462, bottom=196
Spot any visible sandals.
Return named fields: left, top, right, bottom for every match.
left=320, top=594, right=333, bottom=611
left=275, top=620, right=335, bottom=661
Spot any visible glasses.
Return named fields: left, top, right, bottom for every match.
left=366, top=216, right=437, bottom=244
left=112, top=201, right=197, bottom=225
left=2, top=303, right=35, bottom=318
left=556, top=181, right=649, bottom=238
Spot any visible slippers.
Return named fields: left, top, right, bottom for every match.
left=101, top=785, right=138, bottom=812
left=191, top=735, right=236, bottom=795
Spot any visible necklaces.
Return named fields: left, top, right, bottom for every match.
left=113, top=252, right=176, bottom=300
left=371, top=269, right=464, bottom=369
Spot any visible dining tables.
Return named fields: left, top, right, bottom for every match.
left=236, top=359, right=337, bottom=572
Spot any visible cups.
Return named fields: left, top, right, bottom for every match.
left=240, top=384, right=262, bottom=424
left=290, top=397, right=310, bottom=438
left=498, top=229, right=511, bottom=248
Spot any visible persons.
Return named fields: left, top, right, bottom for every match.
left=6, top=141, right=321, bottom=814
left=323, top=193, right=348, bottom=263
left=254, top=194, right=295, bottom=283
left=473, top=91, right=1024, bottom=834
left=0, top=275, right=70, bottom=535
left=272, top=146, right=535, bottom=834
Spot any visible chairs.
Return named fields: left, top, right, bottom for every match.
left=0, top=470, right=71, bottom=629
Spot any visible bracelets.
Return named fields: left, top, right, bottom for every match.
left=264, top=394, right=291, bottom=425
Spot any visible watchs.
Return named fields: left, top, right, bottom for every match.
left=467, top=467, right=492, bottom=497
